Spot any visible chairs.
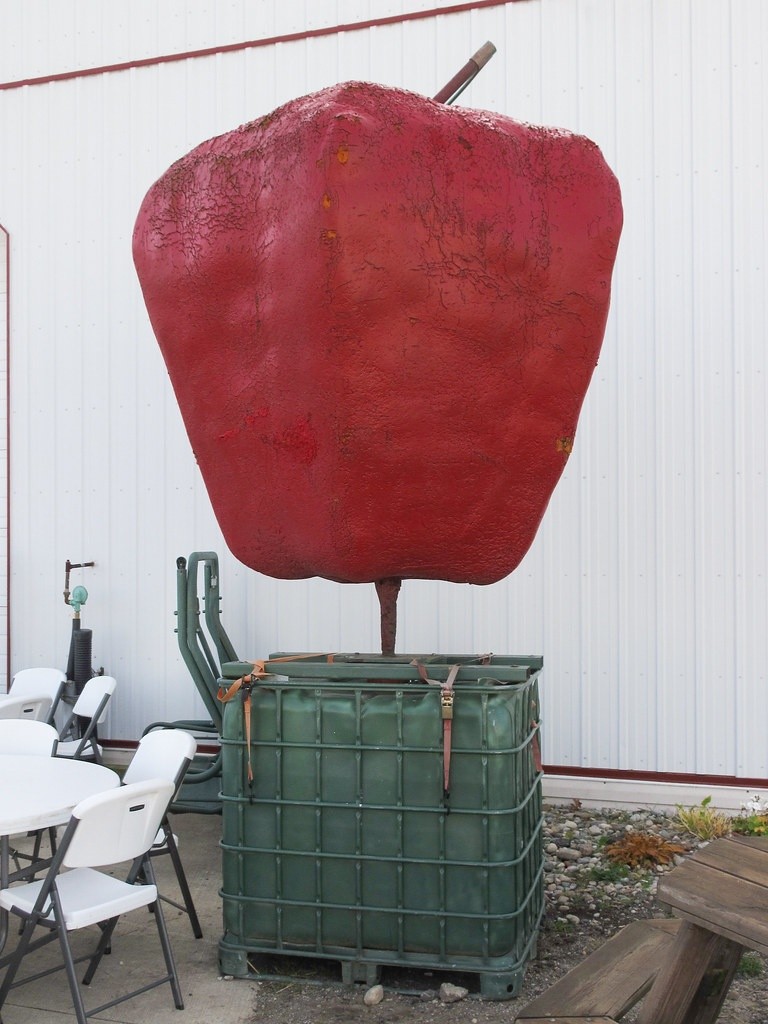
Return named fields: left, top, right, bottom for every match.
left=0, top=668, right=203, bottom=1024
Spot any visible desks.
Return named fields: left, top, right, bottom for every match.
left=1, top=755, right=123, bottom=955
left=636, top=835, right=768, bottom=1024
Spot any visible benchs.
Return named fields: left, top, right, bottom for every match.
left=513, top=919, right=682, bottom=1024
